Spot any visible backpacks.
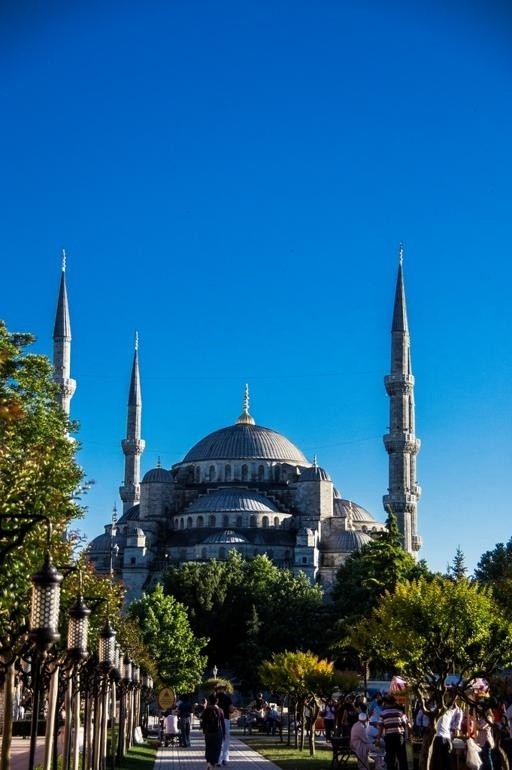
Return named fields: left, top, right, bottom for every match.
left=199, top=706, right=222, bottom=735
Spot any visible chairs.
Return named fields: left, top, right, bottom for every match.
left=324, top=719, right=369, bottom=770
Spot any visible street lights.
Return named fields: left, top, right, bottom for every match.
left=26, top=548, right=63, bottom=769
left=64, top=568, right=154, bottom=770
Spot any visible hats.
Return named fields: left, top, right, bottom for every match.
left=214, top=683, right=227, bottom=692
left=358, top=713, right=367, bottom=721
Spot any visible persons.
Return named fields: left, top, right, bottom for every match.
left=161, top=682, right=281, bottom=770
left=304, top=692, right=512, bottom=770
